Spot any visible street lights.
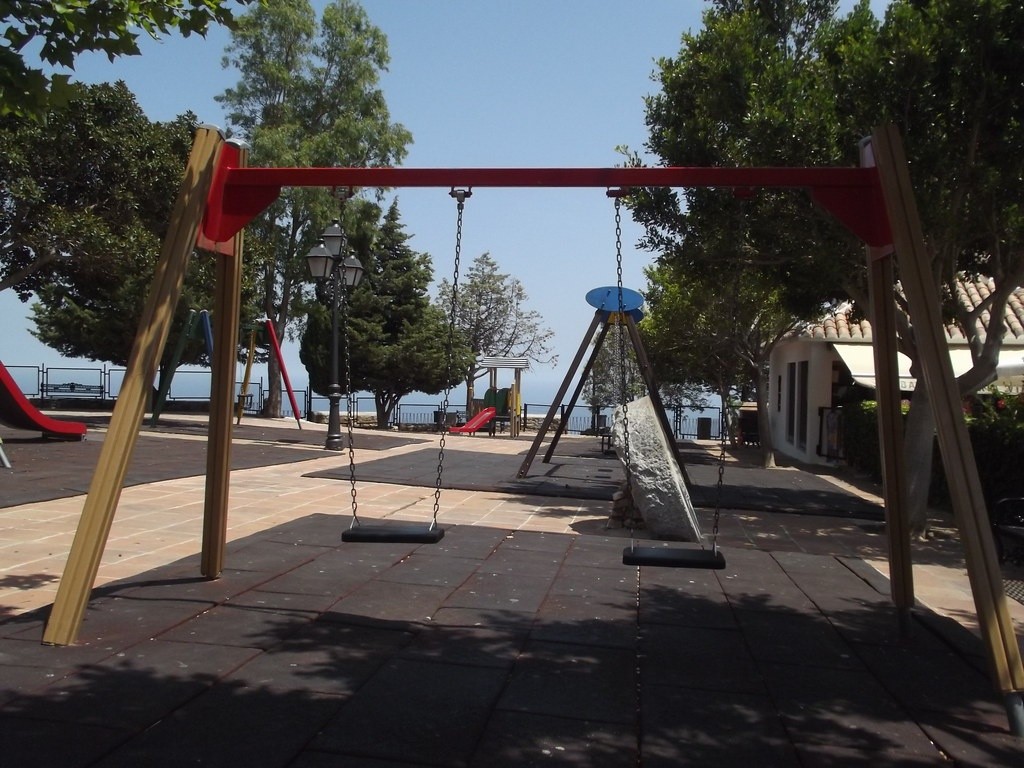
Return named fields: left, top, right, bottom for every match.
left=307, top=215, right=365, bottom=447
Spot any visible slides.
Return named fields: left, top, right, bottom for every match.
left=1, top=361, right=88, bottom=435
left=449, top=408, right=495, bottom=432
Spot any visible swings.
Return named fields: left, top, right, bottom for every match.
left=333, top=186, right=474, bottom=544
left=611, top=197, right=743, bottom=571
